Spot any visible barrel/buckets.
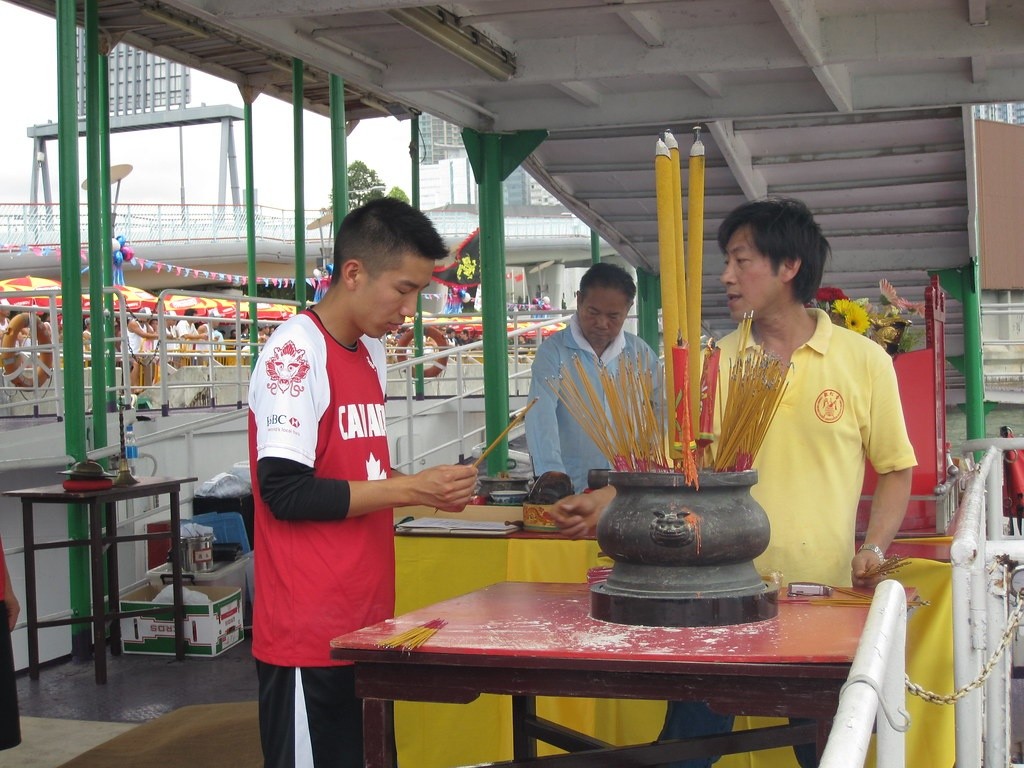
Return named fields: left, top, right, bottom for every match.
left=180, top=534, right=213, bottom=573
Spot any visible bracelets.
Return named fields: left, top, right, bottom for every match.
left=856, top=544, right=885, bottom=565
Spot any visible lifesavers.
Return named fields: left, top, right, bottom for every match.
left=2, top=313, right=53, bottom=393
left=395, top=325, right=449, bottom=379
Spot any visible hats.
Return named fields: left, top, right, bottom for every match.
left=258, top=323, right=272, bottom=328
left=0, top=299, right=11, bottom=306
left=208, top=308, right=225, bottom=317
left=139, top=307, right=151, bottom=315
left=166, top=311, right=176, bottom=317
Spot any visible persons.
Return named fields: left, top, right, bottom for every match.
left=525, top=199, right=918, bottom=768
left=0, top=308, right=280, bottom=396
left=381, top=324, right=532, bottom=362
left=246, top=195, right=480, bottom=768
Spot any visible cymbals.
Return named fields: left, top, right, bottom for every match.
left=56, top=461, right=118, bottom=481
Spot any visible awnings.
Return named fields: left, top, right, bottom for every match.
left=0, top=274, right=317, bottom=323
left=392, top=310, right=566, bottom=336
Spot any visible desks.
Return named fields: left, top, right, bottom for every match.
left=328, top=578, right=917, bottom=768
left=1, top=476, right=199, bottom=683
left=394, top=518, right=958, bottom=768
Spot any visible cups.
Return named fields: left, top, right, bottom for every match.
left=466, top=496, right=485, bottom=505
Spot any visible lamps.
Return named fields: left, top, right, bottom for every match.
left=387, top=4, right=517, bottom=81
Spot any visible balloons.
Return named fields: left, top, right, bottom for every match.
left=313, top=264, right=334, bottom=289
left=112, top=235, right=135, bottom=268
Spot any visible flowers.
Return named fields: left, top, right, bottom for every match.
left=804, top=280, right=926, bottom=358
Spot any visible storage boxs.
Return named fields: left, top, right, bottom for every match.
left=119, top=583, right=245, bottom=660
left=147, top=548, right=254, bottom=616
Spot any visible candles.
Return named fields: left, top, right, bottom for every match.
left=670, top=331, right=697, bottom=451
left=694, top=335, right=721, bottom=450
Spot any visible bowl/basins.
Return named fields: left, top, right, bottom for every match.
left=491, top=491, right=528, bottom=503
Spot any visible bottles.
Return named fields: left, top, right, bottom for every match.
left=124, top=425, right=138, bottom=477
